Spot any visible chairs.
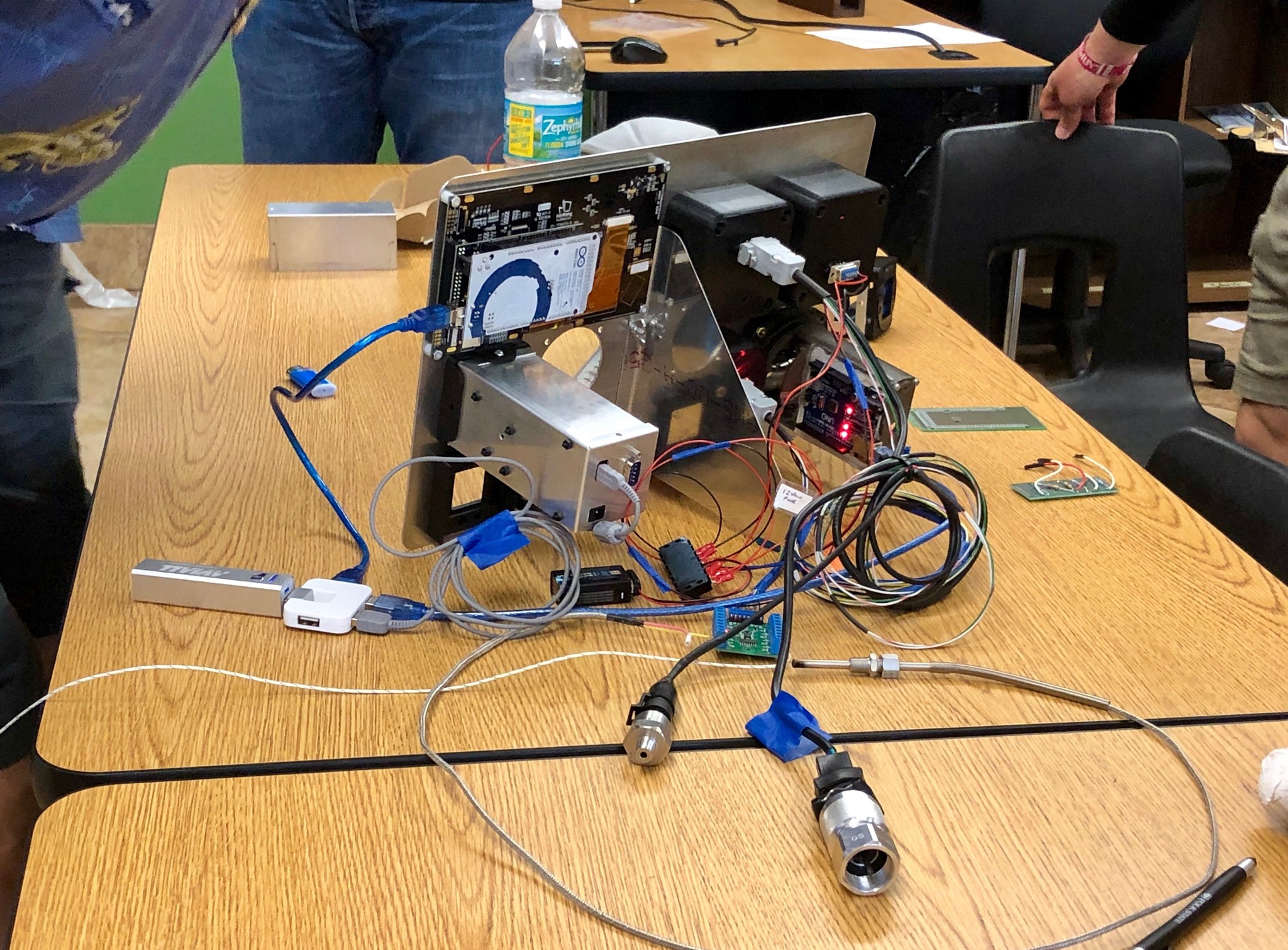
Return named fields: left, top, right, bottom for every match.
left=919, top=121, right=1235, bottom=470
left=978, top=1, right=1236, bottom=389
left=1142, top=427, right=1288, bottom=587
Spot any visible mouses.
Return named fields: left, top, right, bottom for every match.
left=610, top=36, right=668, bottom=68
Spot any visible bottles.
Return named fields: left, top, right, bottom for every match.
left=503, top=0, right=587, bottom=168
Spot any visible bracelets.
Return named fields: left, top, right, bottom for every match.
left=1078, top=33, right=1138, bottom=78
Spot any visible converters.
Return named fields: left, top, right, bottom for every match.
left=550, top=564, right=632, bottom=609
left=657, top=536, right=714, bottom=599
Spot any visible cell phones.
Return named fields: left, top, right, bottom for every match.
left=578, top=41, right=616, bottom=52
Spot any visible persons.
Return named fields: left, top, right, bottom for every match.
left=1038, top=0, right=1288, bottom=470
left=233, top=0, right=536, bottom=164
left=0, top=0, right=263, bottom=950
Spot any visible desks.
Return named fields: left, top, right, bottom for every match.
left=34, top=161, right=1288, bottom=774
left=560, top=0, right=1055, bottom=363
left=11, top=716, right=1287, bottom=949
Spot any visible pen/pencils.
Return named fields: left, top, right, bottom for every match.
left=1129, top=857, right=1257, bottom=950
